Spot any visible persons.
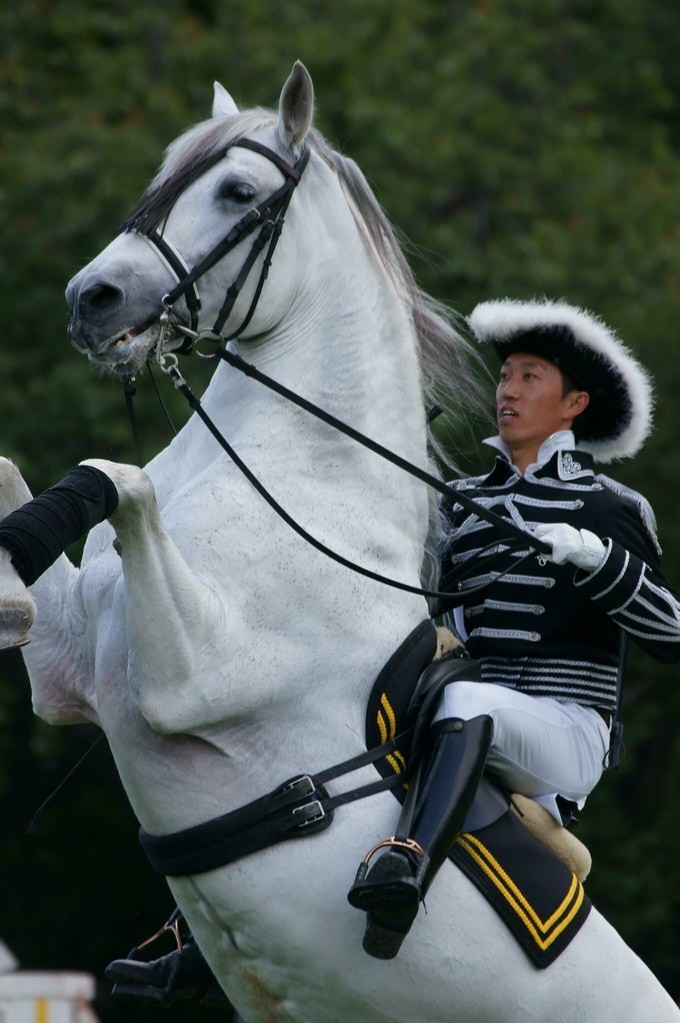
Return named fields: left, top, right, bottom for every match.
left=105, top=300, right=680, bottom=1006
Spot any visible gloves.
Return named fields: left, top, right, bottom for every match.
left=528, top=522, right=606, bottom=573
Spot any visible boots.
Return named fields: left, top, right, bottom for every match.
left=105, top=932, right=232, bottom=1010
left=361, top=714, right=495, bottom=961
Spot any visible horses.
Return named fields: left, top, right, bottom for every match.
left=0, top=59, right=680, bottom=1023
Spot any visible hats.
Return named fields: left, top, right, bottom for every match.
left=463, top=295, right=658, bottom=466
